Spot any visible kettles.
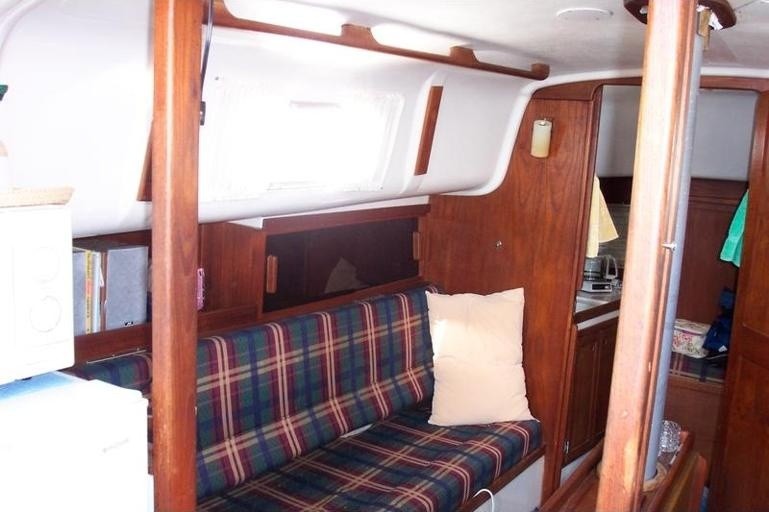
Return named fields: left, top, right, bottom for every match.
left=582, top=254, right=619, bottom=294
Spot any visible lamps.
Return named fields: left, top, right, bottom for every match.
left=530, top=118, right=553, bottom=159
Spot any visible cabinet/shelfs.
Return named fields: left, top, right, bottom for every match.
left=564, top=317, right=621, bottom=463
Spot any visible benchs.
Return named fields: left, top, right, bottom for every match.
left=64, top=283, right=547, bottom=512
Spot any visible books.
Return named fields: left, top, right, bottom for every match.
left=72, top=243, right=150, bottom=334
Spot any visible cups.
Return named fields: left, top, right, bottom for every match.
left=662, top=420, right=680, bottom=451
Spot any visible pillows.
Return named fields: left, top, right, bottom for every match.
left=423, top=287, right=540, bottom=429
